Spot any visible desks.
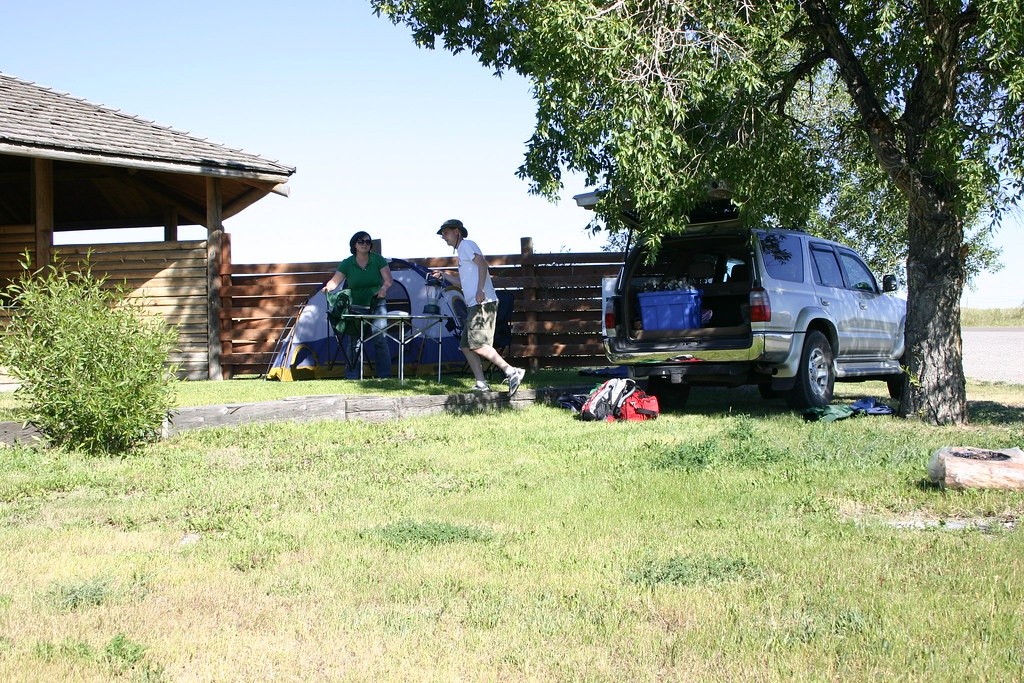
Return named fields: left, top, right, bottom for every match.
left=343, top=313, right=459, bottom=386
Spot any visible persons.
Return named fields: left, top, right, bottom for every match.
left=322, top=231, right=393, bottom=378
left=433, top=219, right=526, bottom=396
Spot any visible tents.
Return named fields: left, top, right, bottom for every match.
left=266, top=257, right=502, bottom=382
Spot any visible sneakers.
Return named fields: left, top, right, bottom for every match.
left=470, top=385, right=492, bottom=391
left=500, top=367, right=526, bottom=396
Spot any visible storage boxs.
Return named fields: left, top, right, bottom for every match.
left=637, top=289, right=704, bottom=331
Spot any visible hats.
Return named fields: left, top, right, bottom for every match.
left=436, top=219, right=468, bottom=238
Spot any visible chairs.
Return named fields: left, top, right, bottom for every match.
left=326, top=288, right=373, bottom=372
left=731, top=263, right=750, bottom=278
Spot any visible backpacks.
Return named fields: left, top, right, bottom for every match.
left=580, top=378, right=658, bottom=421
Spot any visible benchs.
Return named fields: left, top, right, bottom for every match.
left=690, top=279, right=752, bottom=327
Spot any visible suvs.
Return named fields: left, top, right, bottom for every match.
left=572, top=187, right=907, bottom=425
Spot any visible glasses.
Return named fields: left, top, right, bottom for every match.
left=358, top=240, right=371, bottom=245
